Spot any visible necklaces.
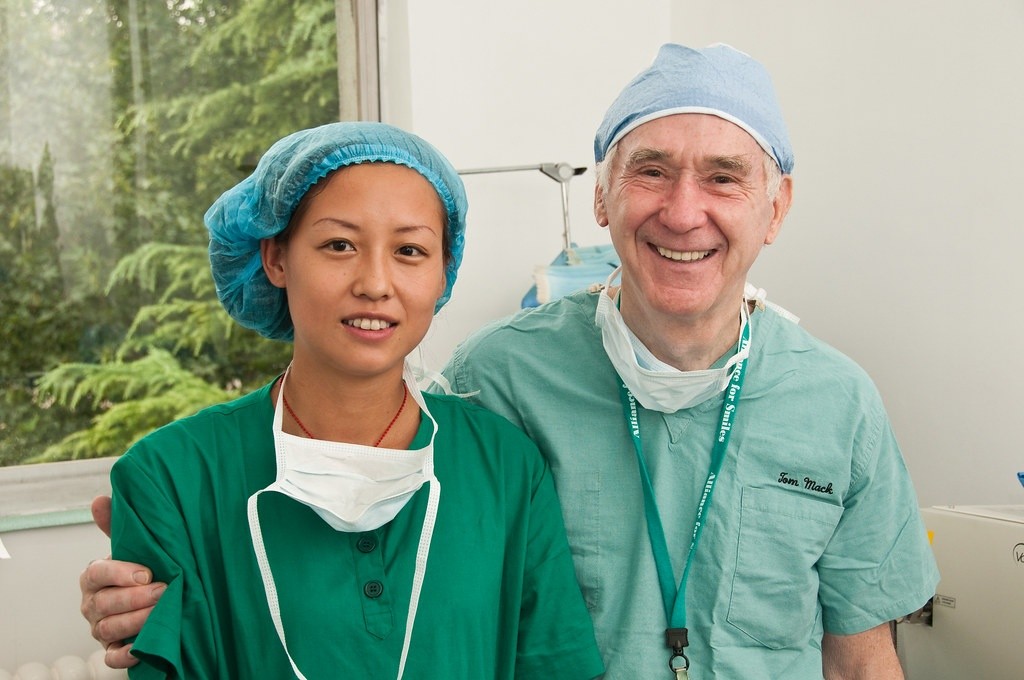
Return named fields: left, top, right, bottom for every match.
left=280, top=378, right=406, bottom=447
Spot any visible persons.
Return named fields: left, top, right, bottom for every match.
left=80, top=43, right=942, bottom=680
left=113, top=120, right=607, bottom=680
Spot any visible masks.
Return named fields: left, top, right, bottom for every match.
left=273, top=430, right=434, bottom=532
left=595, top=289, right=732, bottom=413
